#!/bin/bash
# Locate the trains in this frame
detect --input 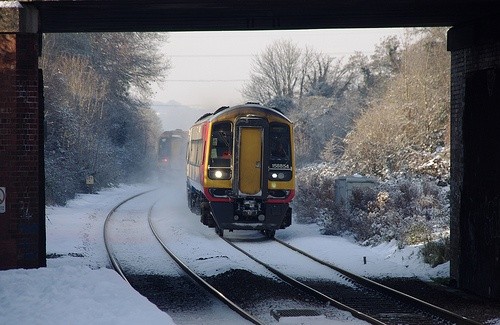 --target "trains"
[186,102,296,241]
[156,129,188,184]
